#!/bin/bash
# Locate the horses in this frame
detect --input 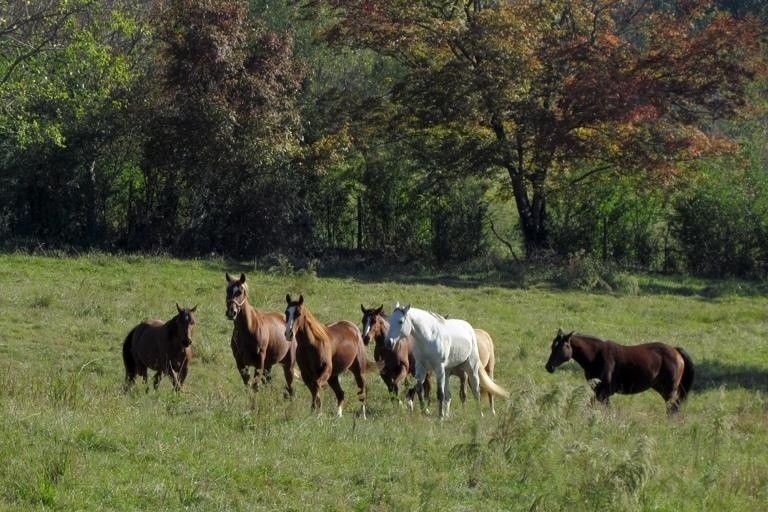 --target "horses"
[544,327,695,420]
[357,300,510,431]
[123,303,199,394]
[284,293,387,421]
[225,271,298,402]
